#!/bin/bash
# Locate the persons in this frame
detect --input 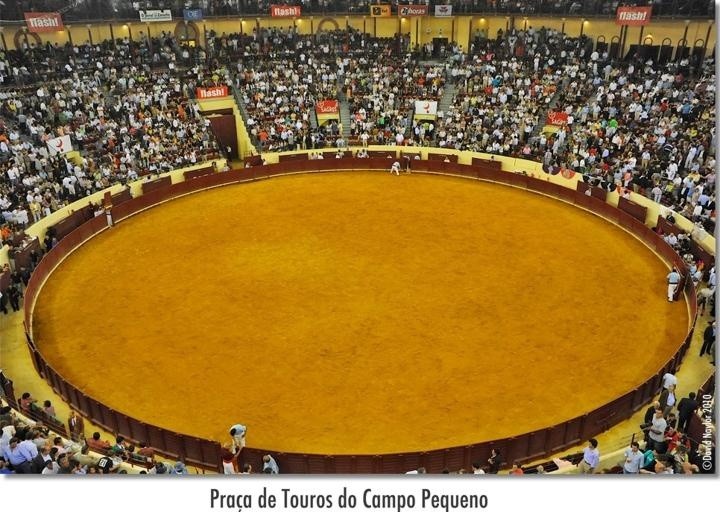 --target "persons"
[0,207,76,324]
[399,372,717,475]
[0,1,717,207]
[1,389,284,473]
[651,208,718,373]
